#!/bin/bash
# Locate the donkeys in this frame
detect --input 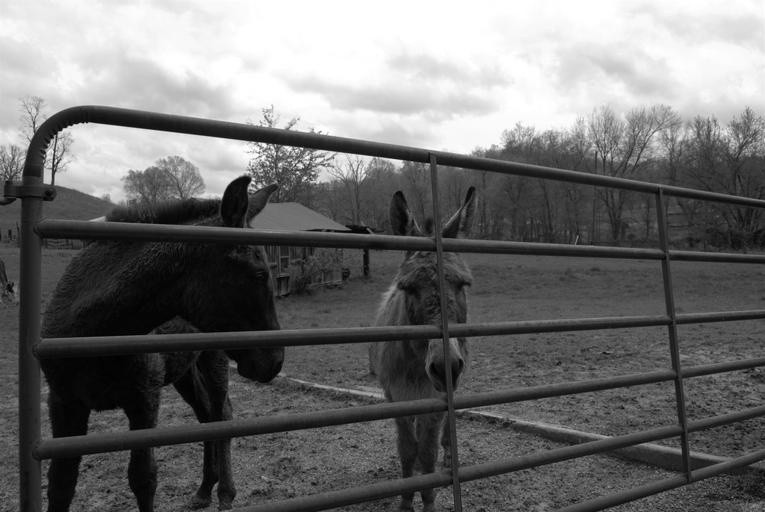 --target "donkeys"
[39,174,286,512]
[367,185,478,512]
